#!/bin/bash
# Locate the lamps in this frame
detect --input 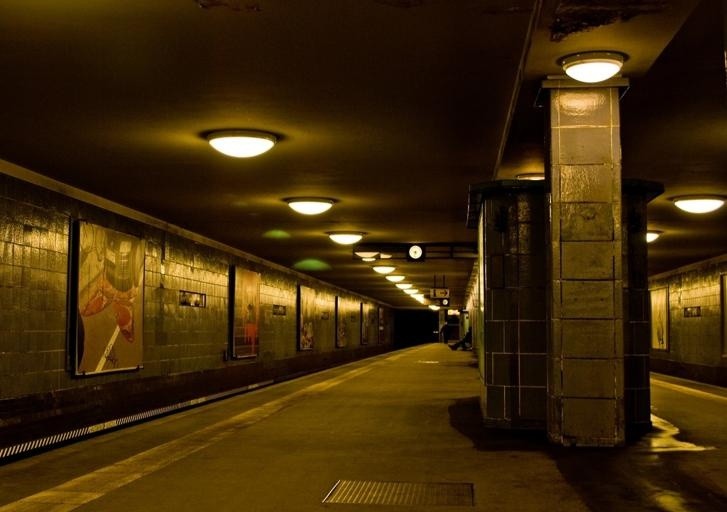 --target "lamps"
[672,196,725,215]
[326,231,364,245]
[206,131,276,158]
[647,230,659,243]
[286,197,335,216]
[559,51,623,84]
[372,265,440,311]
